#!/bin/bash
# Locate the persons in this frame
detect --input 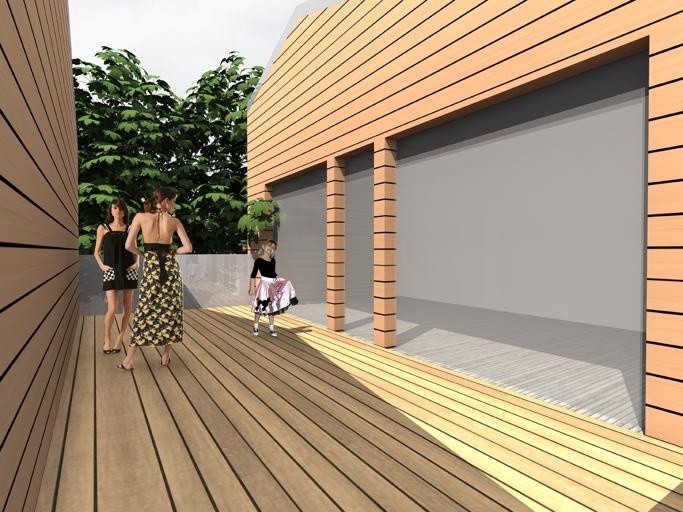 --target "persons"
[92,198,140,355]
[116,186,193,371]
[246,239,297,338]
